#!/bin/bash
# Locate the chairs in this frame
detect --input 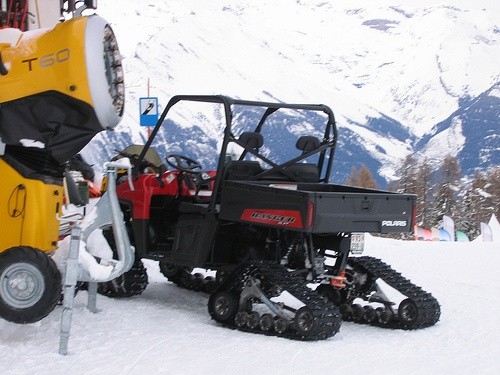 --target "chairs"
[285,136,319,180]
[179,132,264,215]
